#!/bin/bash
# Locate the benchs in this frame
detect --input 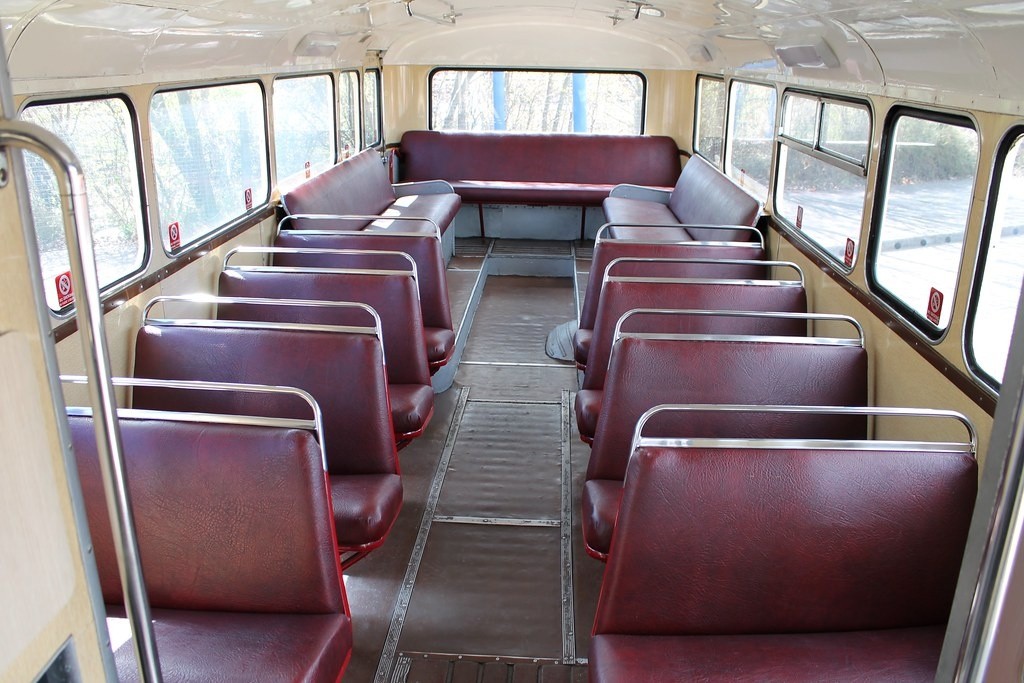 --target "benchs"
[573,222,766,369]
[127,295,405,574]
[273,214,455,380]
[281,146,462,238]
[587,404,979,683]
[389,131,683,246]
[574,255,809,451]
[218,246,436,454]
[603,152,764,241]
[584,308,869,565]
[57,373,354,683]
